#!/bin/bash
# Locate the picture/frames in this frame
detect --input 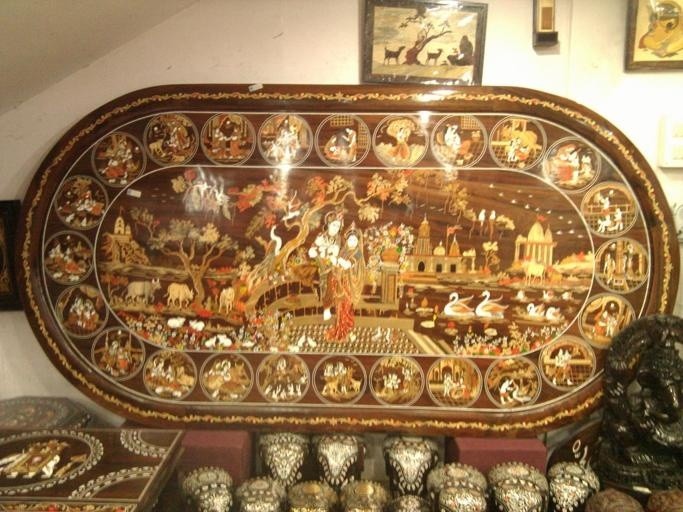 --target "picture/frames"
[623,1,682,75]
[359,1,489,90]
